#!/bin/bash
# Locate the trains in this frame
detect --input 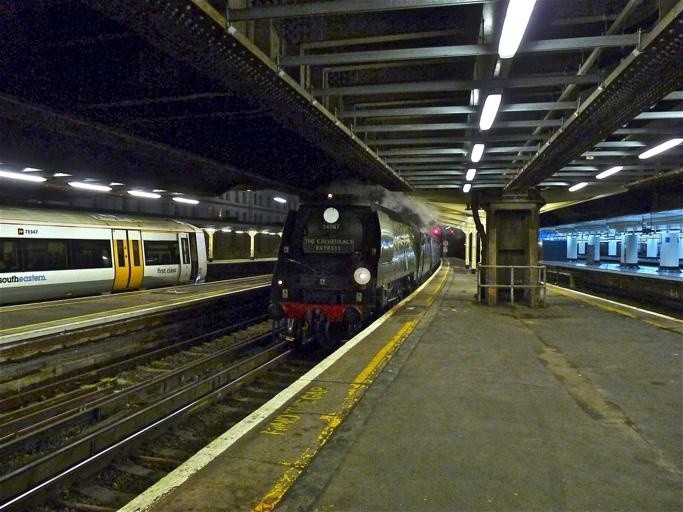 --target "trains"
[271,194,444,348]
[0,206,208,304]
